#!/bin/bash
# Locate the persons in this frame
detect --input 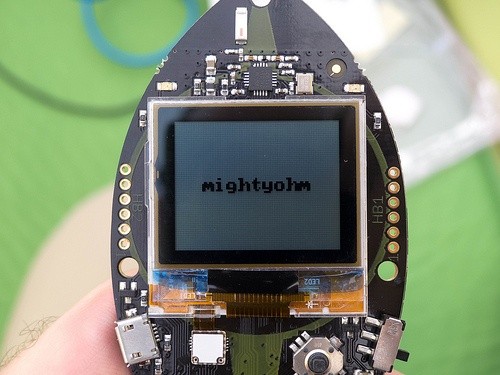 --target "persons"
[1,177,134,375]
